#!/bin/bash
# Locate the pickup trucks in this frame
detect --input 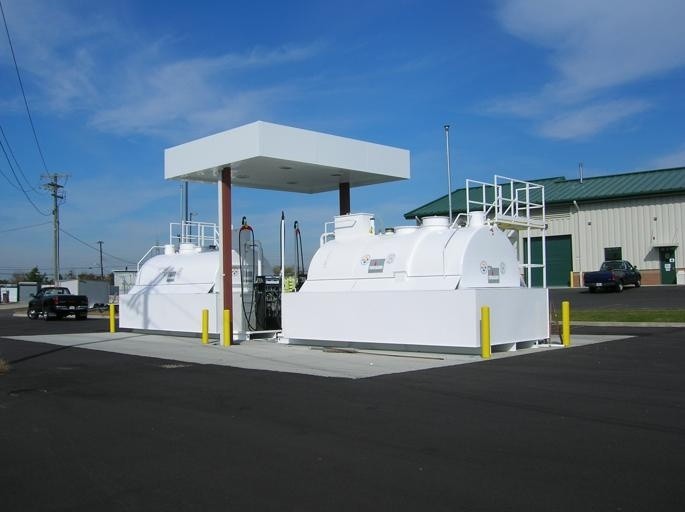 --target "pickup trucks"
[26,286,90,321]
[582,259,643,291]
